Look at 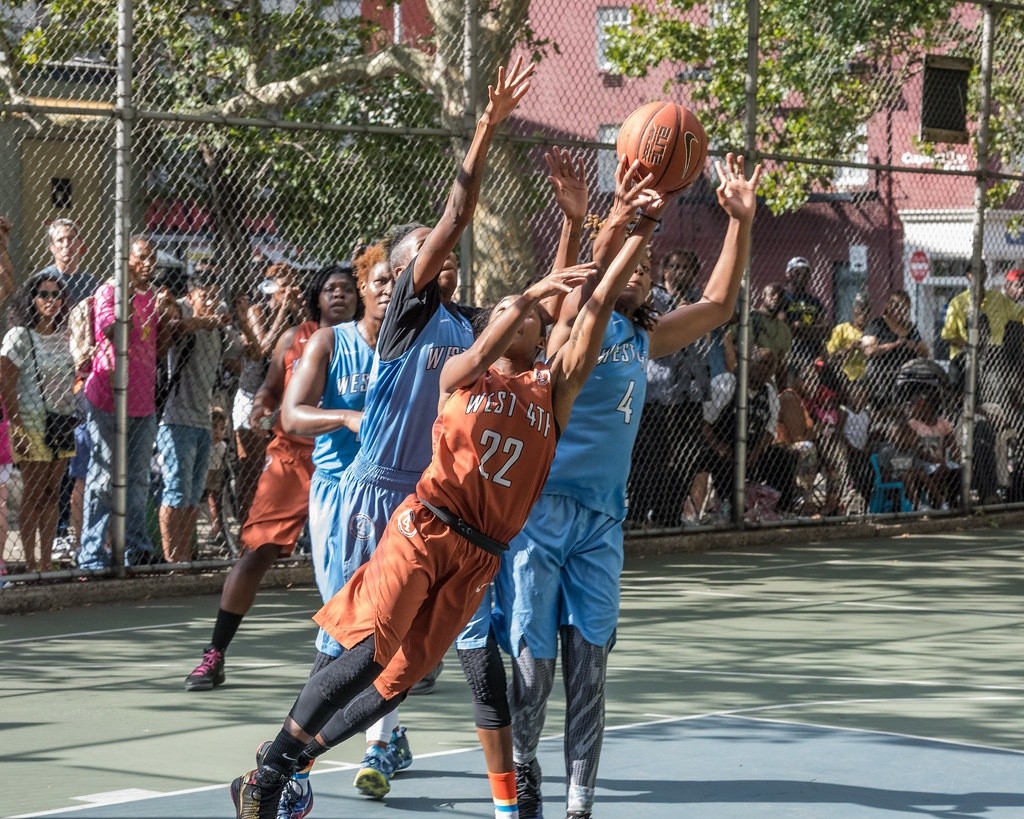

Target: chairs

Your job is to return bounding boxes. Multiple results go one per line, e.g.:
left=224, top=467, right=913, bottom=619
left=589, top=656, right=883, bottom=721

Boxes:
left=868, top=454, right=913, bottom=512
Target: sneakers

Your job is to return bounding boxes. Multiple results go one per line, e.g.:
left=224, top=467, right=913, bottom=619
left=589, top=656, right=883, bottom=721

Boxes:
left=184, top=646, right=226, bottom=690
left=409, top=661, right=443, bottom=696
left=256, top=740, right=296, bottom=819
left=387, top=727, right=413, bottom=778
left=353, top=745, right=394, bottom=798
left=230, top=768, right=289, bottom=819
left=514, top=757, right=544, bottom=819
left=289, top=771, right=315, bottom=818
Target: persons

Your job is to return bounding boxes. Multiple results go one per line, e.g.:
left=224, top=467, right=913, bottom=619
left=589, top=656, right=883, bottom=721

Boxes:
left=484, top=152, right=762, bottom=819
left=230, top=181, right=694, bottom=818
left=280, top=242, right=413, bottom=798
left=622, top=251, right=1024, bottom=530
left=278, top=55, right=588, bottom=819
left=0, top=219, right=304, bottom=575
left=184, top=268, right=443, bottom=695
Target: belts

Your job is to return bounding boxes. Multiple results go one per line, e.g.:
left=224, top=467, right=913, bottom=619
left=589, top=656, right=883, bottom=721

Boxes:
left=422, top=498, right=510, bottom=557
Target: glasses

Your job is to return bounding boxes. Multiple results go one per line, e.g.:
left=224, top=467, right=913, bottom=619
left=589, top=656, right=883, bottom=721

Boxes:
left=37, top=290, right=63, bottom=299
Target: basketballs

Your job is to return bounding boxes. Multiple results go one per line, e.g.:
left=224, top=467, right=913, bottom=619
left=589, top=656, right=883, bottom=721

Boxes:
left=615, top=101, right=708, bottom=192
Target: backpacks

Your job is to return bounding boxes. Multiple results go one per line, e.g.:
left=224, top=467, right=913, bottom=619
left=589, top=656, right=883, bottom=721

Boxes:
left=69, top=279, right=117, bottom=377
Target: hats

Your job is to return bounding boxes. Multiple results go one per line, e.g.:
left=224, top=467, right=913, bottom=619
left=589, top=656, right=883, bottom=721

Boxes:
left=786, top=255, right=810, bottom=274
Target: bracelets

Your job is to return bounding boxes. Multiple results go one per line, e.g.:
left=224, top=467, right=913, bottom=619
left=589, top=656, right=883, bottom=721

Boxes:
left=640, top=213, right=661, bottom=223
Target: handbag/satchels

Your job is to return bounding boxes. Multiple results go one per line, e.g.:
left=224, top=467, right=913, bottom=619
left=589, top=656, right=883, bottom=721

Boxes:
left=44, top=410, right=80, bottom=451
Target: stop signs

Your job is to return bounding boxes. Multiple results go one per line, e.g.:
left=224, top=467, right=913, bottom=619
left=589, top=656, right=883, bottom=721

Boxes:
left=909, top=251, right=931, bottom=284
